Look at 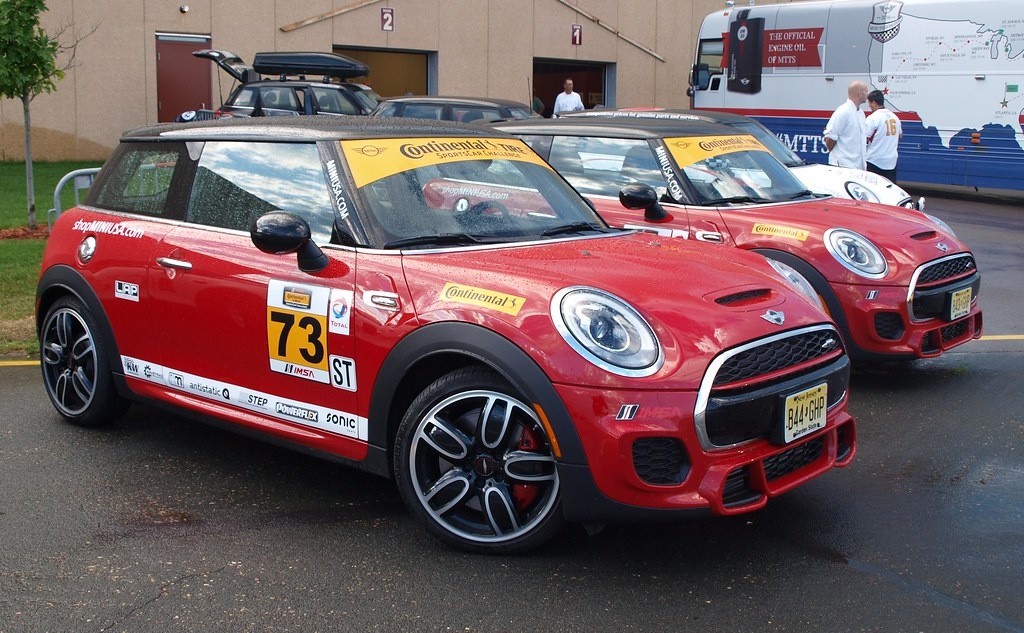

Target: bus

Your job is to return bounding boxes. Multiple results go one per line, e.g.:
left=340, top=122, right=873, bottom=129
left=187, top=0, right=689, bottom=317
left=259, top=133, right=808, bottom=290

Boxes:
left=684, top=1, right=1024, bottom=192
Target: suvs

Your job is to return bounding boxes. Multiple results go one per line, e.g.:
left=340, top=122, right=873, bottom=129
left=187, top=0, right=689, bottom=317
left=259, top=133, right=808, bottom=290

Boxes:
left=33, top=112, right=859, bottom=556
left=556, top=102, right=927, bottom=214
left=173, top=49, right=384, bottom=130
left=469, top=110, right=986, bottom=379
left=368, top=98, right=586, bottom=174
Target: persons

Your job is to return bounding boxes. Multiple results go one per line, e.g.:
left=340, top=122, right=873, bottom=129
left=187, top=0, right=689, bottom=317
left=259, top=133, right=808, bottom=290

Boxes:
left=533, top=88, right=545, bottom=115
left=553, top=78, right=585, bottom=118
left=823, top=80, right=868, bottom=172
left=864, top=90, right=903, bottom=185
